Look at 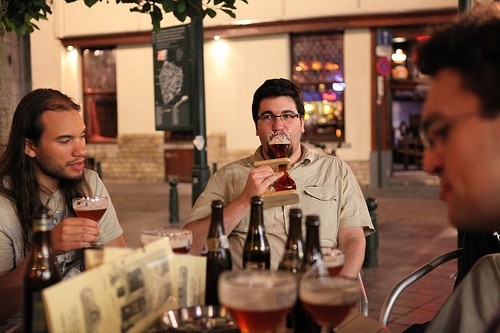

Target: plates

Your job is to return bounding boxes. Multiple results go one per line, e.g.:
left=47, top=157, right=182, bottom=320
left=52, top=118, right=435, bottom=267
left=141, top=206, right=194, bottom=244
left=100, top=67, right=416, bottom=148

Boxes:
left=155, top=304, right=240, bottom=333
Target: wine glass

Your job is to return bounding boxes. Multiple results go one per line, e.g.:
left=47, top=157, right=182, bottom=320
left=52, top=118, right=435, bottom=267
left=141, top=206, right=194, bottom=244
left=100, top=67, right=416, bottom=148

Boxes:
left=71, top=196, right=108, bottom=249
left=267, top=128, right=298, bottom=192
left=299, top=274, right=361, bottom=333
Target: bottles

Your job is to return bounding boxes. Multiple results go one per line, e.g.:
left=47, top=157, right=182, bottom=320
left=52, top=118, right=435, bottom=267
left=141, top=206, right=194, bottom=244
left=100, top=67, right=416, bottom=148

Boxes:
left=242, top=196, right=270, bottom=271
left=276, top=208, right=305, bottom=327
left=299, top=215, right=334, bottom=333
left=23, top=208, right=59, bottom=333
left=201, top=200, right=233, bottom=305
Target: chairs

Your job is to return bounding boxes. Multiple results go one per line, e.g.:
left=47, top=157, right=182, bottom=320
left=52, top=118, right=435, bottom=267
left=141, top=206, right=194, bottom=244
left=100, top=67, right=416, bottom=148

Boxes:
left=379, top=233, right=500, bottom=333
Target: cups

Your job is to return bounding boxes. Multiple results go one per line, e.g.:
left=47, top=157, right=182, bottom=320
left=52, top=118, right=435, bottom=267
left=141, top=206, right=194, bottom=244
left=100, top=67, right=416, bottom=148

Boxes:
left=218, top=270, right=298, bottom=333
left=141, top=226, right=193, bottom=254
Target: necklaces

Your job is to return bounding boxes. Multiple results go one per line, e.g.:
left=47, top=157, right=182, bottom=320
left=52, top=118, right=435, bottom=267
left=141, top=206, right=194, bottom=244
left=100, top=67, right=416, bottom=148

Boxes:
left=46, top=192, right=62, bottom=225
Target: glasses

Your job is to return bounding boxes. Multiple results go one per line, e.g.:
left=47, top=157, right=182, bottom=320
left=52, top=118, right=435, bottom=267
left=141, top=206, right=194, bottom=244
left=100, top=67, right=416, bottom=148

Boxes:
left=257, top=113, right=301, bottom=124
left=418, top=110, right=492, bottom=148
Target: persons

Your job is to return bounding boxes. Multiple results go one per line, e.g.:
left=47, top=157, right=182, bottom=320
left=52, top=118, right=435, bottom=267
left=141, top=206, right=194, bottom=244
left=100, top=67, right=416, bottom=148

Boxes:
left=395, top=120, right=413, bottom=146
left=183, top=78, right=377, bottom=279
left=0, top=87, right=128, bottom=333
left=415, top=1, right=500, bottom=333
